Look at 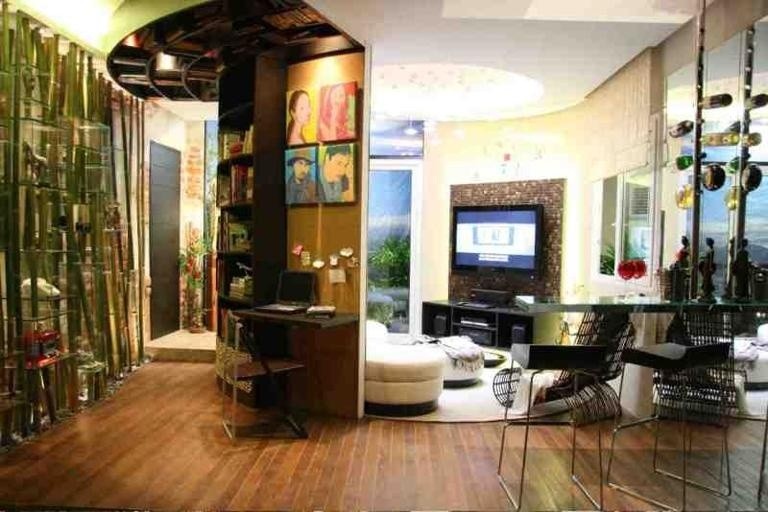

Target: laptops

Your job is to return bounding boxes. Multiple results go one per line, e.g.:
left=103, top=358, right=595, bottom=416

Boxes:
left=255, top=271, right=317, bottom=313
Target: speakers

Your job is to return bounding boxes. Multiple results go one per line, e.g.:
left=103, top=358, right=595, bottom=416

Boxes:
left=511, top=324, right=526, bottom=344
left=433, top=313, right=448, bottom=336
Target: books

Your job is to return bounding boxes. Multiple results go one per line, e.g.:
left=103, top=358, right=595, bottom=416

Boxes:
left=216, top=124, right=253, bottom=300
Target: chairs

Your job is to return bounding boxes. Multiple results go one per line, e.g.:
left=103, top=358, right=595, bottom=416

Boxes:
left=692, top=319, right=768, bottom=391
left=217, top=311, right=311, bottom=442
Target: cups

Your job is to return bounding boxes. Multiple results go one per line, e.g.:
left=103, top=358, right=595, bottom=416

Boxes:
left=660, top=270, right=686, bottom=301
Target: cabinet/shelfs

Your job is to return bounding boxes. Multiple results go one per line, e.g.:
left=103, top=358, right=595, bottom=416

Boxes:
left=217, top=49, right=287, bottom=341
left=421, top=298, right=535, bottom=347
left=1, top=69, right=115, bottom=446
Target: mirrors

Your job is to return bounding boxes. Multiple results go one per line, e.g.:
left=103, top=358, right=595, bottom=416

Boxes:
left=591, top=163, right=654, bottom=289
left=650, top=12, right=768, bottom=423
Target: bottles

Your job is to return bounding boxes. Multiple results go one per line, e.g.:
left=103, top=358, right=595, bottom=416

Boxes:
left=745, top=93, right=768, bottom=111
left=698, top=119, right=763, bottom=175
left=668, top=119, right=704, bottom=138
left=667, top=151, right=708, bottom=174
left=698, top=94, right=732, bottom=109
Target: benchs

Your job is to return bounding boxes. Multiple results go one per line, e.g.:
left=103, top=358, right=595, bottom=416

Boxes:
left=365, top=317, right=445, bottom=416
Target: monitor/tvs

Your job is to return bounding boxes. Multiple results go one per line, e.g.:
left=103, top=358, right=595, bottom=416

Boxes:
left=453, top=205, right=544, bottom=272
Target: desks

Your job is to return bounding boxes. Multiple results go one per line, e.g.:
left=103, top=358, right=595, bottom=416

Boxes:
left=231, top=304, right=359, bottom=330
left=517, top=292, right=768, bottom=316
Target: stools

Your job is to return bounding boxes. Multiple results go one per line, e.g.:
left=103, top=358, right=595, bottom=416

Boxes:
left=420, top=342, right=483, bottom=387
left=602, top=340, right=735, bottom=512
left=498, top=339, right=610, bottom=510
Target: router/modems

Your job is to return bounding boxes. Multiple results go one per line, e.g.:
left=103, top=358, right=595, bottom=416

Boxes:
left=306, top=305, right=337, bottom=317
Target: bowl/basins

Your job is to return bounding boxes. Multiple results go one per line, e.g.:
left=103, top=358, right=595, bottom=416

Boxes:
left=482, top=352, right=506, bottom=368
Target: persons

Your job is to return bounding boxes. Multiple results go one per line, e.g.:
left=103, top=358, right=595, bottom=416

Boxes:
left=318, top=145, right=352, bottom=203
left=318, top=84, right=354, bottom=141
left=733, top=239, right=751, bottom=302
left=286, top=149, right=316, bottom=203
left=286, top=90, right=311, bottom=146
left=677, top=236, right=689, bottom=260
left=698, top=238, right=716, bottom=303
